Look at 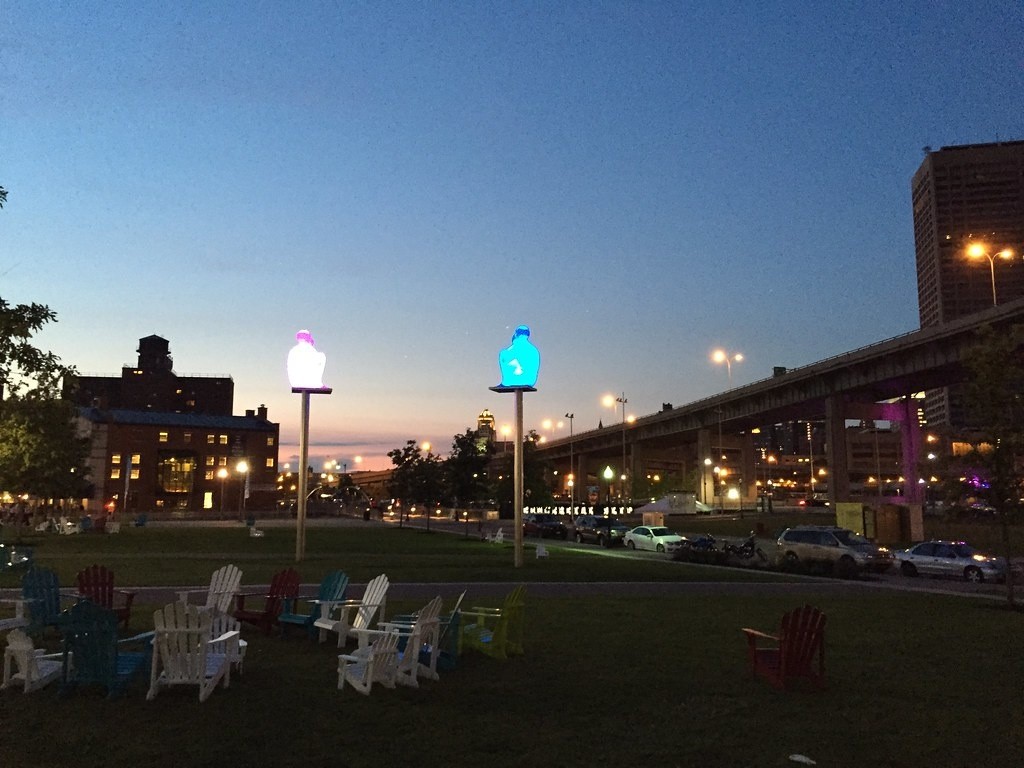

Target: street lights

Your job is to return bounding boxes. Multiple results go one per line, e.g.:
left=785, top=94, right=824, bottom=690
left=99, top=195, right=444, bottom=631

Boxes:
left=968, top=242, right=1014, bottom=309
left=288, top=328, right=332, bottom=564
left=715, top=349, right=742, bottom=392
left=565, top=413, right=574, bottom=524
left=488, top=325, right=541, bottom=570
left=614, top=391, right=628, bottom=520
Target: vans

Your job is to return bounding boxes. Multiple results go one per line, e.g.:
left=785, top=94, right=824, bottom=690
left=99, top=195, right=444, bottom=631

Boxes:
left=776, top=523, right=876, bottom=577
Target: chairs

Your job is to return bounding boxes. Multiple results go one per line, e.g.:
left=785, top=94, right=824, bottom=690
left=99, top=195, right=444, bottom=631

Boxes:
left=145, top=603, right=236, bottom=700
left=277, top=569, right=349, bottom=642
left=77, top=564, right=139, bottom=625
left=336, top=631, right=400, bottom=695
left=54, top=598, right=148, bottom=699
left=313, top=572, right=390, bottom=648
left=353, top=595, right=444, bottom=690
left=392, top=587, right=470, bottom=670
left=450, top=582, right=530, bottom=661
left=198, top=608, right=249, bottom=674
left=536, top=543, right=550, bottom=560
left=486, top=533, right=495, bottom=543
left=22, top=566, right=92, bottom=638
left=36, top=511, right=148, bottom=534
left=0, top=628, right=73, bottom=693
left=176, top=564, right=244, bottom=630
left=742, top=606, right=828, bottom=692
left=232, top=568, right=300, bottom=640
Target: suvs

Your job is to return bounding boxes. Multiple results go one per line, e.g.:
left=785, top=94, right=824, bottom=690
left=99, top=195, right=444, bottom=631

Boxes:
left=574, top=516, right=633, bottom=547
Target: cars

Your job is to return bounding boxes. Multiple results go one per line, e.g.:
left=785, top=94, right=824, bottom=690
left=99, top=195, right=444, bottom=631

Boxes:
left=522, top=513, right=569, bottom=541
left=875, top=540, right=897, bottom=573
left=892, top=539, right=1007, bottom=585
left=624, top=525, right=692, bottom=554
